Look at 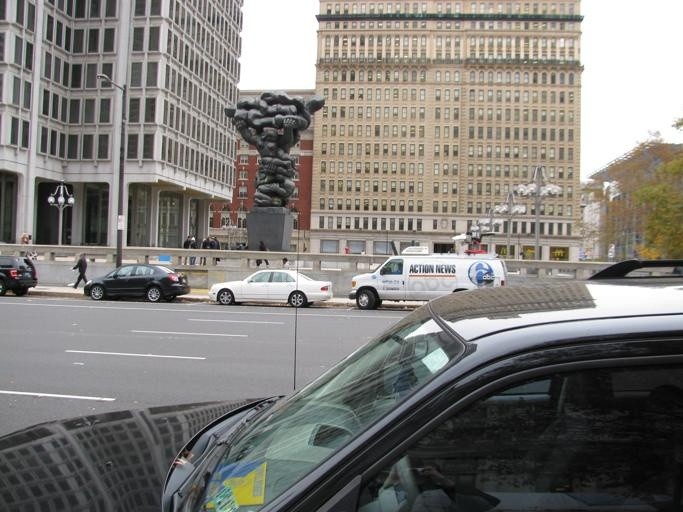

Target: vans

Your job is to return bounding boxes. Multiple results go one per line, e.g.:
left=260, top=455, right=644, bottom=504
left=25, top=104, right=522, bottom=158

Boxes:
left=349, top=245, right=508, bottom=310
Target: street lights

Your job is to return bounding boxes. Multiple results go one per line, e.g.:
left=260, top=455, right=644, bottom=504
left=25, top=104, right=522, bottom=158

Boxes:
left=47, top=180, right=75, bottom=245
left=472, top=192, right=527, bottom=259
left=221, top=218, right=248, bottom=250
left=515, top=164, right=563, bottom=259
left=97, top=73, right=127, bottom=268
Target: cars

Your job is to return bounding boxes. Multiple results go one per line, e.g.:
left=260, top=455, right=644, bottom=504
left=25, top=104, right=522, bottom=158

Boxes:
left=209, top=269, right=332, bottom=308
left=84, top=264, right=190, bottom=302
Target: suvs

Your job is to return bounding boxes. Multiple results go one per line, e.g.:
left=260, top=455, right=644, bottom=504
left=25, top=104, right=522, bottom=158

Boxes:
left=0, top=256, right=37, bottom=296
left=0, top=258, right=683, bottom=511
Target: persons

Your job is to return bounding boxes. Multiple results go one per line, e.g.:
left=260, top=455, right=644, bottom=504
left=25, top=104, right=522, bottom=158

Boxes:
left=281, top=258, right=289, bottom=270
left=184, top=235, right=249, bottom=265
left=26, top=235, right=34, bottom=260
left=72, top=253, right=88, bottom=289
left=257, top=241, right=270, bottom=268
left=385, top=263, right=402, bottom=274
left=20, top=233, right=28, bottom=257
